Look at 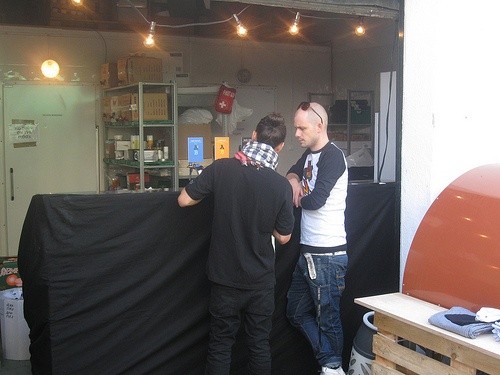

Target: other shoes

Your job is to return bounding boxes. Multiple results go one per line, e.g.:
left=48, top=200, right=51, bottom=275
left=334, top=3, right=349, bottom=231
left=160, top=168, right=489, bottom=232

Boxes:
left=316, top=365, right=346, bottom=375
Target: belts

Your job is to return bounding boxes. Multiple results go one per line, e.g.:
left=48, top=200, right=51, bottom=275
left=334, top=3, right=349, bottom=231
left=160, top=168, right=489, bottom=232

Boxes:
left=299, top=250, right=347, bottom=280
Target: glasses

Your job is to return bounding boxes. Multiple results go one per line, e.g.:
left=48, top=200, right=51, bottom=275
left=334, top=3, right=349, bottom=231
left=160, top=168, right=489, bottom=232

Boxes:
left=296, top=101, right=323, bottom=123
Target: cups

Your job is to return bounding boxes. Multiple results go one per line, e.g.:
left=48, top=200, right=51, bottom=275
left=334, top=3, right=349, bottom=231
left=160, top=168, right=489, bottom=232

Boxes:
left=114, top=135, right=124, bottom=159
left=130, top=135, right=140, bottom=149
left=147, top=136, right=154, bottom=149
left=105, top=139, right=115, bottom=159
left=134, top=151, right=154, bottom=162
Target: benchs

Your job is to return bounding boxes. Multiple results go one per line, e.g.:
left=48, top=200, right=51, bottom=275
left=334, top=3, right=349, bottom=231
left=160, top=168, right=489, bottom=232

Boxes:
left=354, top=161, right=500, bottom=375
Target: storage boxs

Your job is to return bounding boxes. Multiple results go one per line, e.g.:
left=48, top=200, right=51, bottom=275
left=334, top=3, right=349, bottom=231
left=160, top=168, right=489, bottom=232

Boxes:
left=178, top=123, right=213, bottom=160
left=101, top=56, right=168, bottom=121
left=0, top=258, right=23, bottom=291
left=114, top=140, right=173, bottom=191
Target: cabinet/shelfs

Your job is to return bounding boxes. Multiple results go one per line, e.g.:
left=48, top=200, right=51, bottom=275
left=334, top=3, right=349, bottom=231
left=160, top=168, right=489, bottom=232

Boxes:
left=100, top=83, right=178, bottom=193
left=307, top=88, right=375, bottom=186
left=177, top=80, right=228, bottom=188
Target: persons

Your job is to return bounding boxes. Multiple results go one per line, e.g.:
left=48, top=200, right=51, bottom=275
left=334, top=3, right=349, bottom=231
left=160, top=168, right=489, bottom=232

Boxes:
left=178, top=113, right=295, bottom=375
left=286, top=102, right=349, bottom=375
